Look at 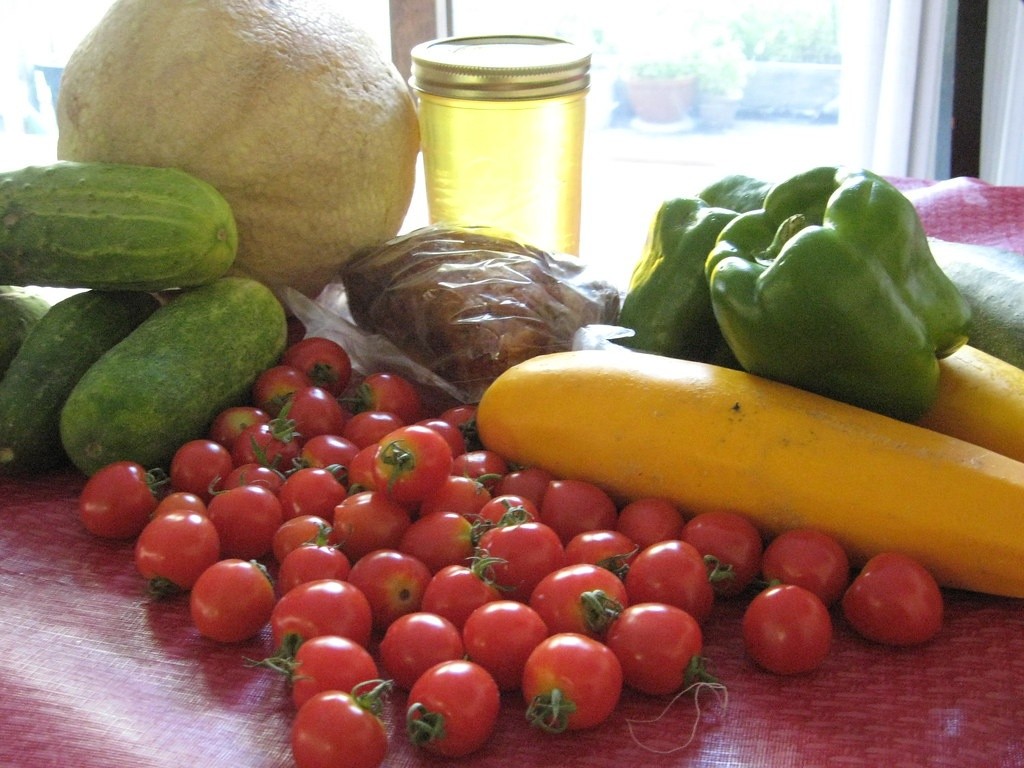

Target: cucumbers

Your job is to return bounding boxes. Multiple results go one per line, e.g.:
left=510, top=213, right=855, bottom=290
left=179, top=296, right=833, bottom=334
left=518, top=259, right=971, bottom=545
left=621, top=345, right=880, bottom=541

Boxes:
left=0, top=161, right=288, bottom=477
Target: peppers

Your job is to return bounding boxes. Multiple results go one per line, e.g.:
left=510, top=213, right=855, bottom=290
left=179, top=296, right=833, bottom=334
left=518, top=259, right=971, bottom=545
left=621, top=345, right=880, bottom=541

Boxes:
left=616, top=165, right=970, bottom=421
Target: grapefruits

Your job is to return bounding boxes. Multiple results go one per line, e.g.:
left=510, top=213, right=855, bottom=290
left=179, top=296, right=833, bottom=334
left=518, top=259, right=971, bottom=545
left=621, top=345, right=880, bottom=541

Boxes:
left=51, top=1, right=427, bottom=307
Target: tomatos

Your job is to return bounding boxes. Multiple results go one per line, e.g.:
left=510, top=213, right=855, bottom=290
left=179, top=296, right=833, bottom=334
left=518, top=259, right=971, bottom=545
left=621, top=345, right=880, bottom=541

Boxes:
left=80, top=337, right=942, bottom=768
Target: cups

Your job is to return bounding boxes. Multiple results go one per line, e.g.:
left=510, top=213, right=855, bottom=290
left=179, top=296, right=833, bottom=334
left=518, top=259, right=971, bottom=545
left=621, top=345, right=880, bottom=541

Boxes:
left=408, top=32, right=594, bottom=265
